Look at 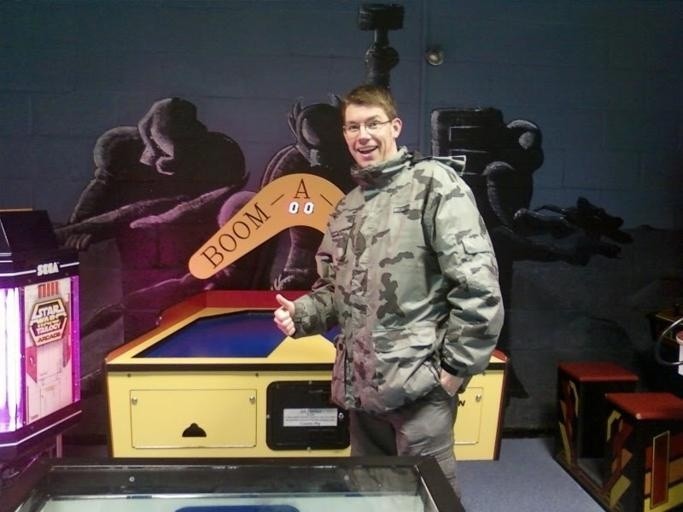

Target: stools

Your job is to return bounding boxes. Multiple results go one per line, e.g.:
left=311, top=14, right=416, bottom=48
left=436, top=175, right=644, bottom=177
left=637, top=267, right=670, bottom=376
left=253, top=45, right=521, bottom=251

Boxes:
left=552, top=353, right=681, bottom=512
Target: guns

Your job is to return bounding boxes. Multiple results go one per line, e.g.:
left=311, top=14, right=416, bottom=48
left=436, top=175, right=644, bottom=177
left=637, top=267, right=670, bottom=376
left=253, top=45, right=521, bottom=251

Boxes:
left=513, top=198, right=634, bottom=258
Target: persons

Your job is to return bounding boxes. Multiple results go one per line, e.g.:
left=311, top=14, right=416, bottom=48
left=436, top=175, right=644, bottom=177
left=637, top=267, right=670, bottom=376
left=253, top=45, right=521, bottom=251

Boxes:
left=272, top=84, right=506, bottom=512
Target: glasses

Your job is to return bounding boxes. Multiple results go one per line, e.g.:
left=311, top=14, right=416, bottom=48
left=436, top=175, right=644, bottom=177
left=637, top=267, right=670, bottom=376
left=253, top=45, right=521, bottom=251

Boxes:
left=344, top=120, right=391, bottom=138
